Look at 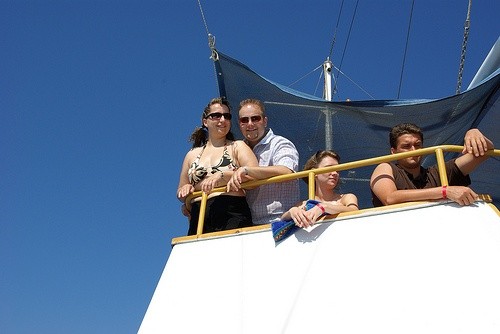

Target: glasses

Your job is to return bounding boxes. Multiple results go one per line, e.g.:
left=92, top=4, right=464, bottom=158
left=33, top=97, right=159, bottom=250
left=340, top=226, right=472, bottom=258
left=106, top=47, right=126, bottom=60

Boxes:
left=238, top=115, right=266, bottom=123
left=205, top=112, right=232, bottom=120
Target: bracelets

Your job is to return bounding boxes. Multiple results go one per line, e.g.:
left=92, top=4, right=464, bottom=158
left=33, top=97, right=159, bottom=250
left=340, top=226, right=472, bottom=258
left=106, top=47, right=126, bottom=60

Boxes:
left=220, top=171, right=225, bottom=180
left=243, top=167, right=248, bottom=175
left=316, top=204, right=325, bottom=214
left=442, top=186, right=446, bottom=200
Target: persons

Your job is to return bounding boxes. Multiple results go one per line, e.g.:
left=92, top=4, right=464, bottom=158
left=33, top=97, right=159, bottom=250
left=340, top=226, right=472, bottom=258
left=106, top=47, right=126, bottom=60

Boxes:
left=280, top=151, right=358, bottom=229
left=369, top=123, right=494, bottom=207
left=176, top=99, right=259, bottom=236
left=181, top=99, right=300, bottom=226
